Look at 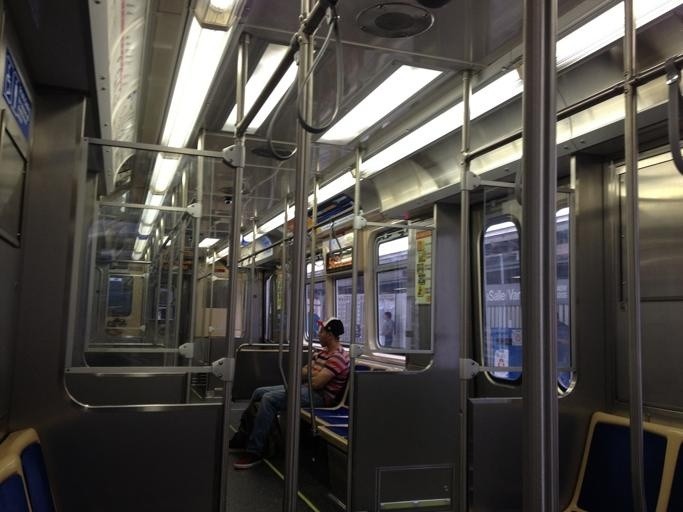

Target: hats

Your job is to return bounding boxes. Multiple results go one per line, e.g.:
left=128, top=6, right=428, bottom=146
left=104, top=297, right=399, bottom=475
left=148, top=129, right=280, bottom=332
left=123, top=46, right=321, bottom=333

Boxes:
left=316, top=317, right=344, bottom=337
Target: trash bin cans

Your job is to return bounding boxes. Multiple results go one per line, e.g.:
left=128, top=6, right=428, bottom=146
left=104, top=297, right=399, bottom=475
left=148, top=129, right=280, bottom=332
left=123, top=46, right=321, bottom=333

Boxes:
left=492, top=327, right=523, bottom=381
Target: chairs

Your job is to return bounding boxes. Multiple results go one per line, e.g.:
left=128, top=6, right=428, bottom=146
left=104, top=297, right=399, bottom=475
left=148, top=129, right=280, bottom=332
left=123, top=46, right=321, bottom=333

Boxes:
left=299, top=356, right=405, bottom=452
left=559, top=410, right=682, bottom=507
left=0, top=425, right=61, bottom=510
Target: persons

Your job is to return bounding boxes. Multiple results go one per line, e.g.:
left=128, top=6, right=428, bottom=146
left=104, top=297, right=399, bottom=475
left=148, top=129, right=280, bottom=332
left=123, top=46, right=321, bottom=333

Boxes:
left=230, top=317, right=351, bottom=469
left=380, top=311, right=393, bottom=346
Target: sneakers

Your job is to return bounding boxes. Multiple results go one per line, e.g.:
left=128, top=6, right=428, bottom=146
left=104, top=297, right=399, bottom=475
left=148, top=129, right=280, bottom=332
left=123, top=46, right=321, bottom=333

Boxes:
left=228, top=434, right=262, bottom=469
